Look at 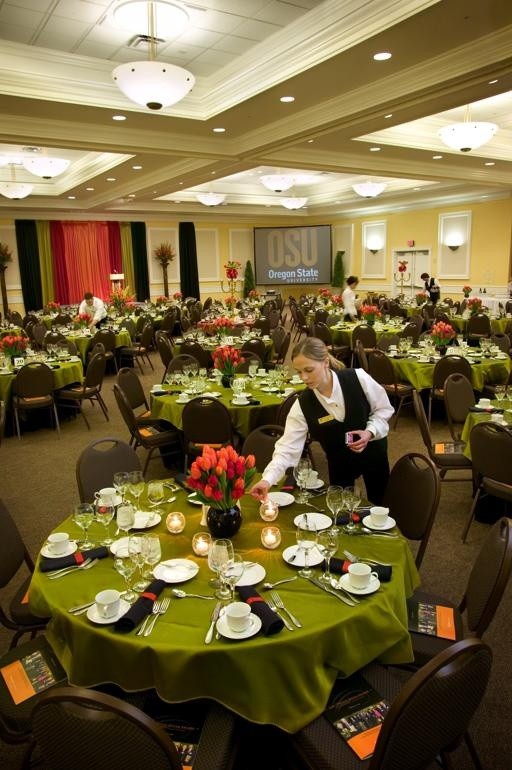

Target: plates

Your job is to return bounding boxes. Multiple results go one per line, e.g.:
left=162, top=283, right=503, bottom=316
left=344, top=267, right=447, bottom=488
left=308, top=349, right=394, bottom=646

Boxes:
left=213, top=612, right=263, bottom=642
left=338, top=571, right=383, bottom=598
left=361, top=514, right=396, bottom=531
left=230, top=398, right=251, bottom=405
left=86, top=599, right=131, bottom=626
left=472, top=404, right=494, bottom=410
left=175, top=398, right=193, bottom=404
left=39, top=541, right=77, bottom=559
left=485, top=420, right=509, bottom=426
left=288, top=379, right=305, bottom=384
left=150, top=390, right=166, bottom=393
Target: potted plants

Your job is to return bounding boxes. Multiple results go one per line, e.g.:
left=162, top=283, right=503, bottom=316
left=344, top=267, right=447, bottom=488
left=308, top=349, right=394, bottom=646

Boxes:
left=331, top=250, right=345, bottom=296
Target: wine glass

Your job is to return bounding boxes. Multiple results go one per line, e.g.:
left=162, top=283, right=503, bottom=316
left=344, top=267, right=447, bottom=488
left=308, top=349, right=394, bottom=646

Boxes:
left=0, top=342, right=69, bottom=368
left=495, top=384, right=512, bottom=413
left=167, top=361, right=290, bottom=402
left=73, top=457, right=364, bottom=607
left=395, top=334, right=508, bottom=365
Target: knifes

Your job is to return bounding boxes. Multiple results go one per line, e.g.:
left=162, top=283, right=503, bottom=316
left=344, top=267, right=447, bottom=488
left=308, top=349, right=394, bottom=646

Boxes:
left=204, top=598, right=221, bottom=649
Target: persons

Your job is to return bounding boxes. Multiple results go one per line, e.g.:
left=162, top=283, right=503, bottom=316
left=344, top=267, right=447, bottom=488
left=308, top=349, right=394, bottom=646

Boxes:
left=250, top=336, right=396, bottom=508
left=78, top=292, right=107, bottom=330
left=342, top=276, right=360, bottom=322
left=421, top=273, right=441, bottom=306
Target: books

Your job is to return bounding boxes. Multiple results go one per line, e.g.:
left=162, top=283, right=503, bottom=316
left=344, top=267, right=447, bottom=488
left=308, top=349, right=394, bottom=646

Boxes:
left=140, top=698, right=210, bottom=770
left=22, top=396, right=46, bottom=401
left=138, top=422, right=174, bottom=438
left=0, top=647, right=68, bottom=706
left=141, top=410, right=152, bottom=417
left=389, top=383, right=407, bottom=391
left=322, top=671, right=392, bottom=761
left=406, top=599, right=456, bottom=640
left=434, top=443, right=466, bottom=455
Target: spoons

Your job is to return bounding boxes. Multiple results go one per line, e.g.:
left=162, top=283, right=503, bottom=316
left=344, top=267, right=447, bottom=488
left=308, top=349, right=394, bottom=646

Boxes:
left=358, top=527, right=398, bottom=538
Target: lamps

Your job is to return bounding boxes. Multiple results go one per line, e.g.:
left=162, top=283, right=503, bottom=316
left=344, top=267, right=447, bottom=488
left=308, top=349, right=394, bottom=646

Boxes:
left=438, top=103, right=499, bottom=154
left=110, top=0, right=196, bottom=113
left=195, top=173, right=388, bottom=211
left=367, top=233, right=384, bottom=255
left=444, top=230, right=465, bottom=251
left=0, top=150, right=34, bottom=203
left=24, top=145, right=71, bottom=184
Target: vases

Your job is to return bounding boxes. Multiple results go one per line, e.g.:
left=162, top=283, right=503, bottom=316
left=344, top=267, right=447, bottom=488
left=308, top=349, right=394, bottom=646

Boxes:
left=206, top=507, right=243, bottom=538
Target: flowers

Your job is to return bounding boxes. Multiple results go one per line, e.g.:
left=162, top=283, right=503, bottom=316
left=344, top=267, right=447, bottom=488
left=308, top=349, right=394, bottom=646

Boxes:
left=398, top=260, right=409, bottom=272
left=462, top=286, right=472, bottom=294
left=223, top=260, right=243, bottom=280
left=103, top=283, right=135, bottom=309
left=248, top=289, right=258, bottom=298
left=152, top=238, right=177, bottom=263
left=187, top=444, right=263, bottom=512
left=317, top=288, right=328, bottom=295
left=0, top=241, right=14, bottom=267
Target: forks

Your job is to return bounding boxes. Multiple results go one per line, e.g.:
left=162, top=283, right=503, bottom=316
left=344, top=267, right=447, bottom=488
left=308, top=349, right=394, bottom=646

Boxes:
left=265, top=591, right=303, bottom=632
left=135, top=597, right=172, bottom=642
left=45, top=556, right=100, bottom=580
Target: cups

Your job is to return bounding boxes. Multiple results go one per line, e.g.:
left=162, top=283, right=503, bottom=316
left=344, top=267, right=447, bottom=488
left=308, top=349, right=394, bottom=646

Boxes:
left=96, top=589, right=121, bottom=617
left=368, top=505, right=391, bottom=527
left=347, top=562, right=379, bottom=589
left=47, top=533, right=69, bottom=555
left=153, top=384, right=162, bottom=391
left=293, top=375, right=300, bottom=381
left=478, top=399, right=492, bottom=408
left=285, top=387, right=298, bottom=398
left=492, top=413, right=504, bottom=424
left=223, top=601, right=253, bottom=631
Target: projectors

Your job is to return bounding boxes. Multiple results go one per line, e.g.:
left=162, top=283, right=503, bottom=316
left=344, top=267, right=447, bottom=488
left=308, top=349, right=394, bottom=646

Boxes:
left=266, top=290, right=276, bottom=294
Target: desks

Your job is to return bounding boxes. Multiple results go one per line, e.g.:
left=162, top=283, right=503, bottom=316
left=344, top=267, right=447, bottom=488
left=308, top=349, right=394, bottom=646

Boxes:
left=29, top=473, right=419, bottom=732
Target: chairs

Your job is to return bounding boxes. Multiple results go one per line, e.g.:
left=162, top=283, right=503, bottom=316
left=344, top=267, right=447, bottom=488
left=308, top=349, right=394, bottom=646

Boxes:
left=242, top=426, right=305, bottom=479
left=384, top=517, right=512, bottom=668
left=1, top=293, right=511, bottom=479
left=77, top=438, right=142, bottom=503
left=0, top=501, right=49, bottom=654
left=382, top=452, right=441, bottom=571
left=0, top=635, right=69, bottom=744
left=460, top=421, right=512, bottom=542
left=290, top=640, right=495, bottom=768
left=17, top=688, right=235, bottom=770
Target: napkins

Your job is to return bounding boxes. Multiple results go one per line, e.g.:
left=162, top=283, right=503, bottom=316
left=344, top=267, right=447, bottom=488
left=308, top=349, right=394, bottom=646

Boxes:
left=282, top=475, right=296, bottom=511
left=234, top=586, right=285, bottom=636
left=39, top=546, right=108, bottom=573
left=113, top=579, right=165, bottom=632
left=175, top=475, right=193, bottom=494
left=322, top=558, right=394, bottom=581
left=335, top=510, right=371, bottom=525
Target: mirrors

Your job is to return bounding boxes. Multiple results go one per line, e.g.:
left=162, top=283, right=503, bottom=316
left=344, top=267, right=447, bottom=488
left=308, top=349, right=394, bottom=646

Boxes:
left=359, top=219, right=388, bottom=280
left=436, top=209, right=472, bottom=280
